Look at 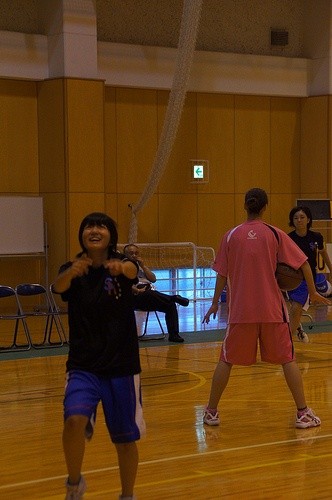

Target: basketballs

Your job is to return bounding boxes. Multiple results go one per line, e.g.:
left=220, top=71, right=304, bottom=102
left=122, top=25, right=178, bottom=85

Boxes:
left=275, top=263, right=303, bottom=290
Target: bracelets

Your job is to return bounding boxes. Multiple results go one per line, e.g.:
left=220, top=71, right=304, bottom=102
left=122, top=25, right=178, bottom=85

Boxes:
left=212, top=300, right=219, bottom=303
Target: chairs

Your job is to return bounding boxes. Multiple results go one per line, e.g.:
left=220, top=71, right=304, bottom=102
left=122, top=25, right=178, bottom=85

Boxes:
left=0, top=283, right=165, bottom=353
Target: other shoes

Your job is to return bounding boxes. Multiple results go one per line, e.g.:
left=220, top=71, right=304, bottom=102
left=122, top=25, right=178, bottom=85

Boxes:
left=64, top=474, right=86, bottom=500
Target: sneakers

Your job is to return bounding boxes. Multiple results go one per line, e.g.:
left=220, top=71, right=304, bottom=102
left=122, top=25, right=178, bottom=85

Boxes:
left=203, top=408, right=220, bottom=425
left=296, top=408, right=321, bottom=428
left=296, top=323, right=309, bottom=344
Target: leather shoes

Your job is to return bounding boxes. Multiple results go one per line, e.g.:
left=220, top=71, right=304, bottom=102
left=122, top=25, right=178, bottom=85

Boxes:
left=174, top=295, right=189, bottom=306
left=168, top=335, right=184, bottom=343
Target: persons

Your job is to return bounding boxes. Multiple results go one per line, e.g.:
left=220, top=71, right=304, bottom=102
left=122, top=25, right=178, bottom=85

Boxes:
left=276, top=206, right=332, bottom=343
left=123, top=244, right=189, bottom=344
left=202, top=187, right=330, bottom=428
left=51, top=212, right=156, bottom=500
